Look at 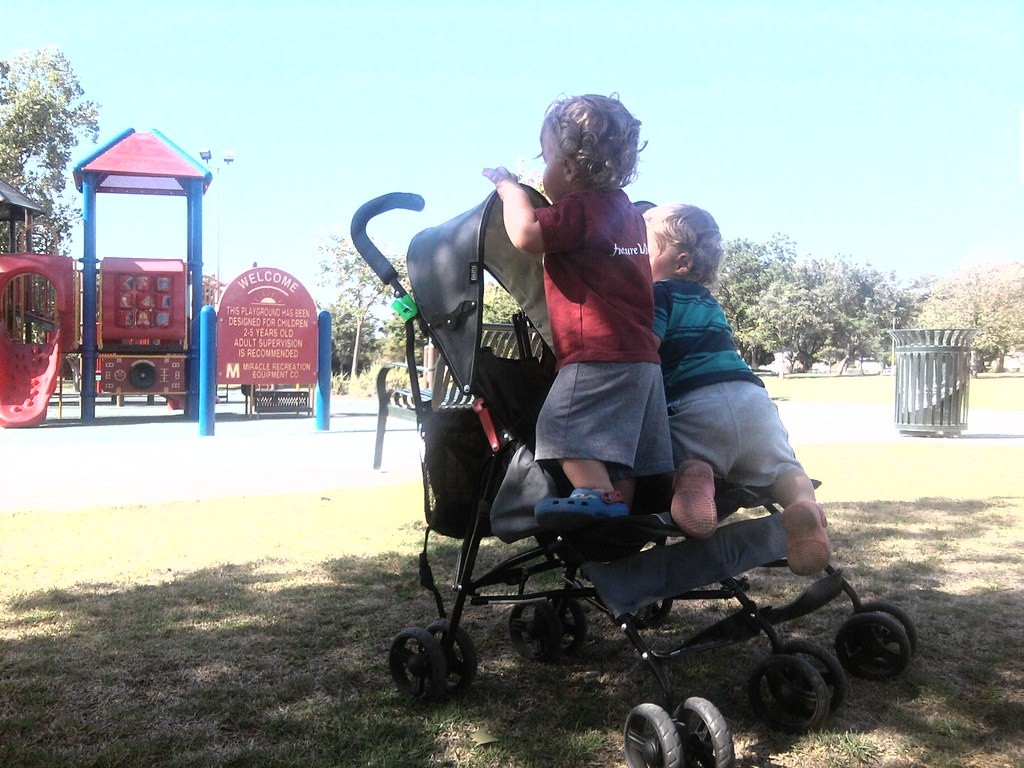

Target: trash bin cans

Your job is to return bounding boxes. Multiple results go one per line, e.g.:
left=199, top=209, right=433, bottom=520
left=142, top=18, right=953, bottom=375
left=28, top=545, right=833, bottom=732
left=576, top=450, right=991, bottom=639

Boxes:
left=886, top=327, right=982, bottom=438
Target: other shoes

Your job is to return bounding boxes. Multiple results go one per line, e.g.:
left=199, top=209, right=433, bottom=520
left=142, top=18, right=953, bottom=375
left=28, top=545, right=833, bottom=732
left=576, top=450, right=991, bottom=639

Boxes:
left=671, top=460, right=717, bottom=539
left=782, top=499, right=831, bottom=577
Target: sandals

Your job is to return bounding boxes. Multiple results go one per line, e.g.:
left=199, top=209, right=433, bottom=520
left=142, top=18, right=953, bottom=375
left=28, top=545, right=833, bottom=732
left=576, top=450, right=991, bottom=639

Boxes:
left=534, top=489, right=629, bottom=520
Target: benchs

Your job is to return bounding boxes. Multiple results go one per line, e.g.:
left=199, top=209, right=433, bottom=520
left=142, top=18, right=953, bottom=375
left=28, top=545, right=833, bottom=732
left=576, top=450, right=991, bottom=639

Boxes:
left=372, top=325, right=542, bottom=470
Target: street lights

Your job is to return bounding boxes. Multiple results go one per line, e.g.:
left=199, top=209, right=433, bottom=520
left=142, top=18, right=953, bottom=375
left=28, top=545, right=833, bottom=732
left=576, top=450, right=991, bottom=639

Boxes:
left=200, top=149, right=235, bottom=306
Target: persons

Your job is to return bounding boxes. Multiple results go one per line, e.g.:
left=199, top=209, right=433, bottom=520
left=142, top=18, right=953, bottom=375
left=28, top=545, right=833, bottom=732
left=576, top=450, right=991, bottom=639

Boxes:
left=480, top=92, right=676, bottom=525
left=633, top=200, right=833, bottom=575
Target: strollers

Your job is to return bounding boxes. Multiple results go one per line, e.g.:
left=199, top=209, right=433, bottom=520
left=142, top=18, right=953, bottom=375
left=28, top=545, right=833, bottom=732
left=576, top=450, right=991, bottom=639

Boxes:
left=349, top=184, right=917, bottom=767
